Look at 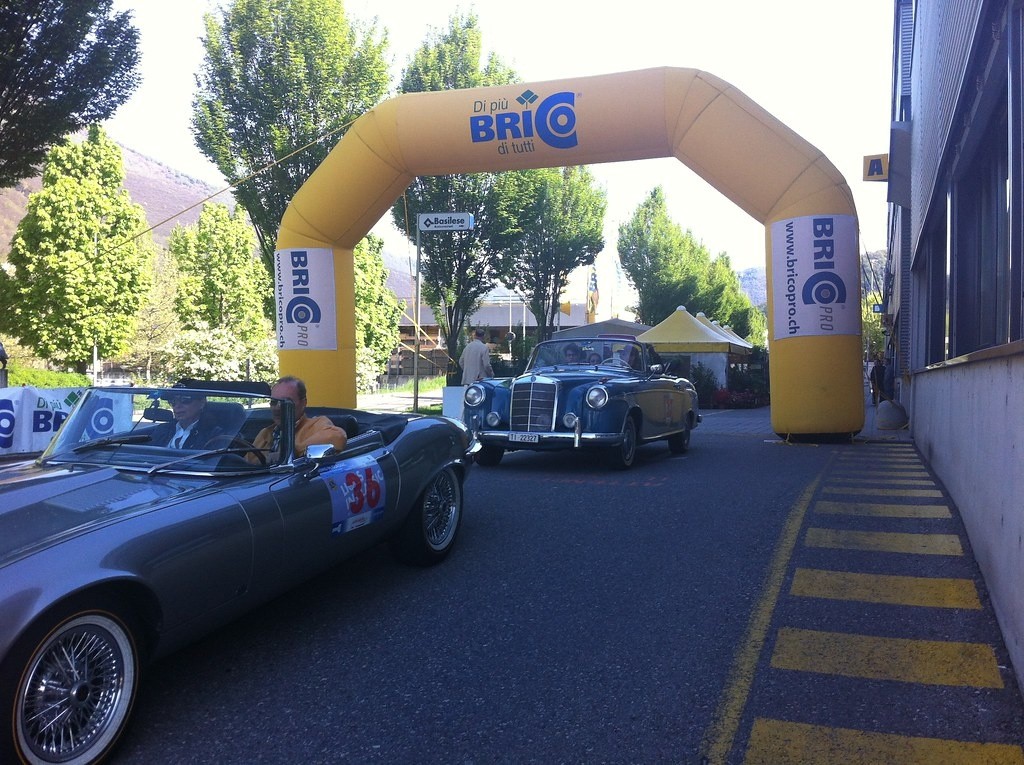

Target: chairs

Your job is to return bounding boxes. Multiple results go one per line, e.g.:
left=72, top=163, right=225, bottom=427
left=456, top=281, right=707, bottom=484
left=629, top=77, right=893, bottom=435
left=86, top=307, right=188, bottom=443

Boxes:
left=201, top=402, right=248, bottom=453
left=317, top=414, right=361, bottom=441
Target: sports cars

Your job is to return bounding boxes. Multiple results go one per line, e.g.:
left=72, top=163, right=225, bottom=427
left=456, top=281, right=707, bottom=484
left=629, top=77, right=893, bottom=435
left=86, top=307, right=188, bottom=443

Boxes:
left=0, top=378, right=485, bottom=765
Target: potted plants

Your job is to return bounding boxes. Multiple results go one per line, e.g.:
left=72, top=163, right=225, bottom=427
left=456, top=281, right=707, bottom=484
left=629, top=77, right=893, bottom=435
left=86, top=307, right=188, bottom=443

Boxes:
left=688, top=344, right=771, bottom=409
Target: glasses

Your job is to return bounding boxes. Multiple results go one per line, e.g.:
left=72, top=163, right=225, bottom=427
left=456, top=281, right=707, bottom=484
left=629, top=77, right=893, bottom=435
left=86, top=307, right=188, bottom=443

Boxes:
left=567, top=354, right=578, bottom=357
left=268, top=398, right=302, bottom=406
left=590, top=359, right=600, bottom=362
left=167, top=395, right=197, bottom=406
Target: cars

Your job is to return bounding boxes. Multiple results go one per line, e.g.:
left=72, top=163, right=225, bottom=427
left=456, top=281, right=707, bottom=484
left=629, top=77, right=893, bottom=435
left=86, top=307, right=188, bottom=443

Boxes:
left=460, top=337, right=705, bottom=468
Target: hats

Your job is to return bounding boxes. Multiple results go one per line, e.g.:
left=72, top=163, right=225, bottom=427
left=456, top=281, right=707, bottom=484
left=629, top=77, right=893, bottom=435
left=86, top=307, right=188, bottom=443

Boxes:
left=161, top=379, right=204, bottom=400
left=617, top=346, right=639, bottom=356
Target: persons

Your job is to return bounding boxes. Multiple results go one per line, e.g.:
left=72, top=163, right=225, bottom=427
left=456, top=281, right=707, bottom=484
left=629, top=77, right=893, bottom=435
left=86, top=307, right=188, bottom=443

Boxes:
left=244, top=375, right=347, bottom=466
left=870, top=359, right=886, bottom=406
left=564, top=344, right=582, bottom=364
left=616, top=345, right=641, bottom=370
left=459, top=329, right=495, bottom=386
left=590, top=353, right=602, bottom=365
left=144, top=377, right=227, bottom=452
left=883, top=358, right=894, bottom=401
